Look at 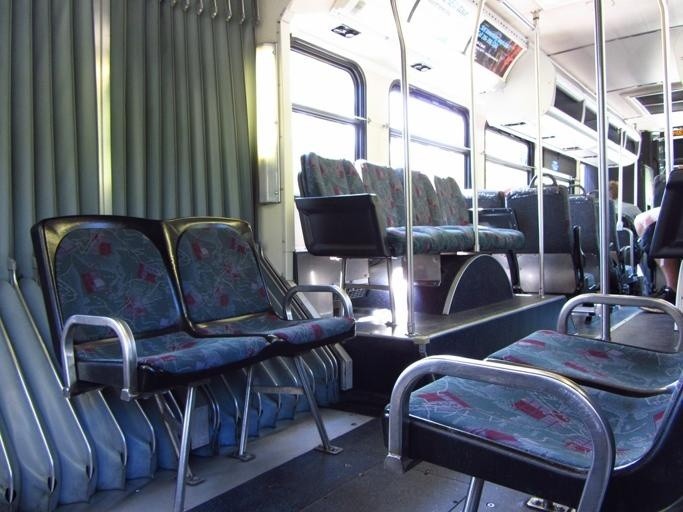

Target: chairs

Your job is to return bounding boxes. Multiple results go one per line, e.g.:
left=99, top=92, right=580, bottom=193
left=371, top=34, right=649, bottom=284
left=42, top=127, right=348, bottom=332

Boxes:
left=460, top=188, right=506, bottom=209
left=508, top=174, right=576, bottom=254
left=567, top=186, right=600, bottom=255
left=31, top=215, right=290, bottom=511
left=400, top=168, right=506, bottom=252
left=355, top=158, right=458, bottom=252
left=587, top=189, right=620, bottom=252
left=488, top=292, right=683, bottom=394
left=301, top=150, right=432, bottom=256
left=164, top=217, right=356, bottom=459
left=382, top=355, right=683, bottom=508
left=435, top=175, right=526, bottom=252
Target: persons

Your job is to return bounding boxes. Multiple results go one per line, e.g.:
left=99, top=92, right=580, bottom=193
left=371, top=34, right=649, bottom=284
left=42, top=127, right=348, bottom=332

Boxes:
left=608, top=180, right=642, bottom=220
left=635, top=206, right=679, bottom=313
